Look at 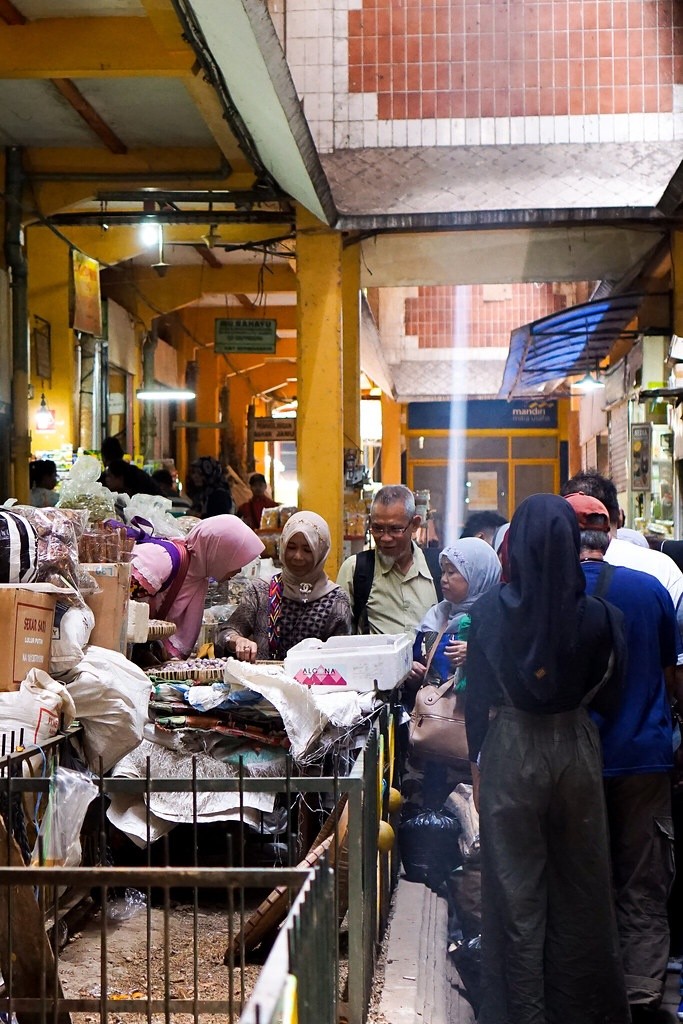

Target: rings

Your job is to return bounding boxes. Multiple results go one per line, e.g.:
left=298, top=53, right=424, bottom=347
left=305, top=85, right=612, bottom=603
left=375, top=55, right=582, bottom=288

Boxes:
left=244, top=646, right=249, bottom=648
left=458, top=657, right=460, bottom=663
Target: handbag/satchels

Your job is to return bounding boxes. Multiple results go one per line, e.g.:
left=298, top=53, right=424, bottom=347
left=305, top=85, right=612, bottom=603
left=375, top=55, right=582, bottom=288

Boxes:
left=408, top=682, right=470, bottom=762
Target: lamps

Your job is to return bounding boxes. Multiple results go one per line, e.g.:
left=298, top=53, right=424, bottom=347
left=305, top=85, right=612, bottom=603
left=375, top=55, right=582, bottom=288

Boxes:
left=134, top=387, right=196, bottom=402
left=571, top=319, right=606, bottom=389
left=35, top=376, right=54, bottom=429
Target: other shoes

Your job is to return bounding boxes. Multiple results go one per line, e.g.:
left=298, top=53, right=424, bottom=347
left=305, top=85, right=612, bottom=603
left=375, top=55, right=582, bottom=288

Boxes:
left=667, top=957, right=683, bottom=969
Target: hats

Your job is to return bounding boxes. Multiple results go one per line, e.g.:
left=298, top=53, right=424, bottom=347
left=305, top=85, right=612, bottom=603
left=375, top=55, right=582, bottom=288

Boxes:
left=562, top=492, right=611, bottom=535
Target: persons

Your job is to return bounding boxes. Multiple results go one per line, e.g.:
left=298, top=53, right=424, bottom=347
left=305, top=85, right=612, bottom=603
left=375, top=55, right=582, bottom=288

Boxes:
left=335, top=485, right=443, bottom=635
left=561, top=473, right=683, bottom=1024
left=464, top=494, right=624, bottom=1024
left=213, top=511, right=354, bottom=664
left=131, top=513, right=266, bottom=670
left=460, top=512, right=512, bottom=561
left=236, top=473, right=280, bottom=530
left=184, top=457, right=232, bottom=519
left=29, top=460, right=60, bottom=506
left=96, top=436, right=188, bottom=507
left=401, top=537, right=501, bottom=937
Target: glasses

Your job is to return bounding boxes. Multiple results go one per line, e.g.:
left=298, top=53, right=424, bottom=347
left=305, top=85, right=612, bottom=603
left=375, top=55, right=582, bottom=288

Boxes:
left=366, top=512, right=415, bottom=537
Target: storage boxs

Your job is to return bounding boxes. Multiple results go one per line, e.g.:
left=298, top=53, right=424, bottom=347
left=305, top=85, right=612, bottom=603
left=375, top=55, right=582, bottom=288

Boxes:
left=0, top=563, right=148, bottom=693
left=285, top=632, right=417, bottom=692
left=641, top=336, right=674, bottom=390
